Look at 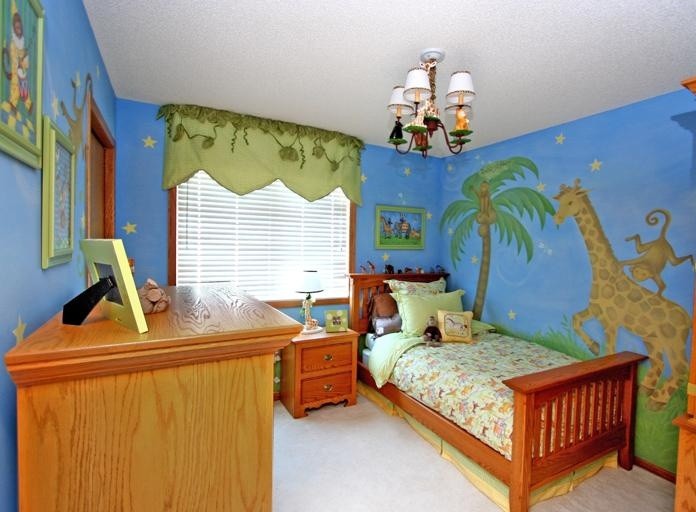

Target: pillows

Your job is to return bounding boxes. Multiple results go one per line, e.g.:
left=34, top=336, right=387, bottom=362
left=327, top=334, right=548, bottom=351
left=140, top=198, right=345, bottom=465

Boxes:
left=371, top=278, right=475, bottom=345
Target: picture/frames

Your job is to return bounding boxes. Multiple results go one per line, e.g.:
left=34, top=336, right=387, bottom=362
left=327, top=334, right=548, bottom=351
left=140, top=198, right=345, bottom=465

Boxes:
left=1, top=0, right=148, bottom=334
left=375, top=204, right=425, bottom=251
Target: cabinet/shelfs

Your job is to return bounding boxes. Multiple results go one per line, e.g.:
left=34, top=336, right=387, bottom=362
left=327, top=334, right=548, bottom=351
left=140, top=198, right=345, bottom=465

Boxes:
left=672, top=412, right=696, bottom=512
left=4, top=284, right=361, bottom=512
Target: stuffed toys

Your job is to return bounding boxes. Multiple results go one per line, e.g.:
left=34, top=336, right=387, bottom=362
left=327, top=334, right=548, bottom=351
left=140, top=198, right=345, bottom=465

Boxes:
left=422, top=316, right=441, bottom=343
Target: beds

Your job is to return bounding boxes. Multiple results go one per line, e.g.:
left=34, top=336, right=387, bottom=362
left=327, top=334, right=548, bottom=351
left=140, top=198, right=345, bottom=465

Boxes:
left=347, top=271, right=650, bottom=512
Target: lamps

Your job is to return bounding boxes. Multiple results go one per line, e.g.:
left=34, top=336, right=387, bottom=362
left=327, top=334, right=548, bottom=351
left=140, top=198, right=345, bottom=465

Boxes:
left=386, top=51, right=476, bottom=158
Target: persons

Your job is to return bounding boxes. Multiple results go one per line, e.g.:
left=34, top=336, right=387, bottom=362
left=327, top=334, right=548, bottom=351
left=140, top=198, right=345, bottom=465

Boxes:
left=7, top=13, right=35, bottom=116
